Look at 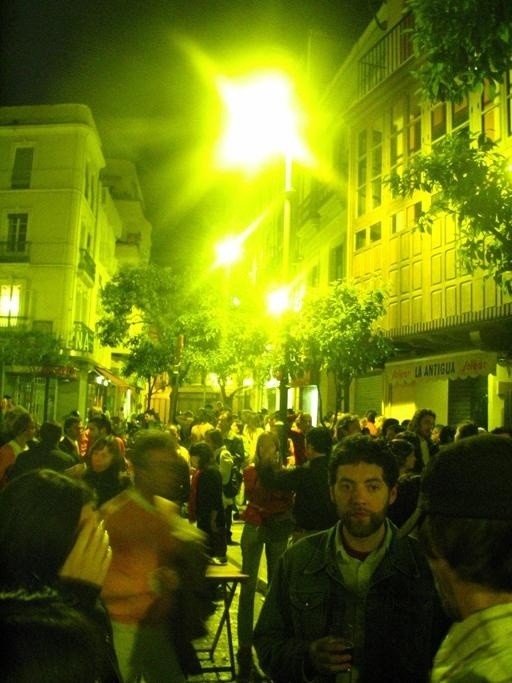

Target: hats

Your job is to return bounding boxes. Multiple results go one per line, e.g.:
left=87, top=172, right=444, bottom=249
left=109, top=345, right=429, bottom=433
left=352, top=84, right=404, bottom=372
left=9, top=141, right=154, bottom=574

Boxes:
left=398, top=433, right=512, bottom=539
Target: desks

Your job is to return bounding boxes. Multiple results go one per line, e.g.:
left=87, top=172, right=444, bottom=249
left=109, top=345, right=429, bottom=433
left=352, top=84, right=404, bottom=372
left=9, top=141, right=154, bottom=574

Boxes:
left=189, top=558, right=256, bottom=682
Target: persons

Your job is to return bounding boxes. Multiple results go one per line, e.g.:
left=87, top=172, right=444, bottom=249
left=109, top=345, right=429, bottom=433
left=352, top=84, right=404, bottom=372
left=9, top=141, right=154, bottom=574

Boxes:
left=0, top=389, right=511, bottom=683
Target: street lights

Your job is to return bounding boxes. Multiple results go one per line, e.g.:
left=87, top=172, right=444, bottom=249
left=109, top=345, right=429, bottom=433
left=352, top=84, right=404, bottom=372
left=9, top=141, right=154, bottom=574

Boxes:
left=210, top=53, right=291, bottom=467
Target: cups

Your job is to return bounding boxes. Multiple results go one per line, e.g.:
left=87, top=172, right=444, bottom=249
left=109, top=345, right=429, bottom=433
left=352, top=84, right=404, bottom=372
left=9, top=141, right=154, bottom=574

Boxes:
left=326, top=621, right=355, bottom=672
